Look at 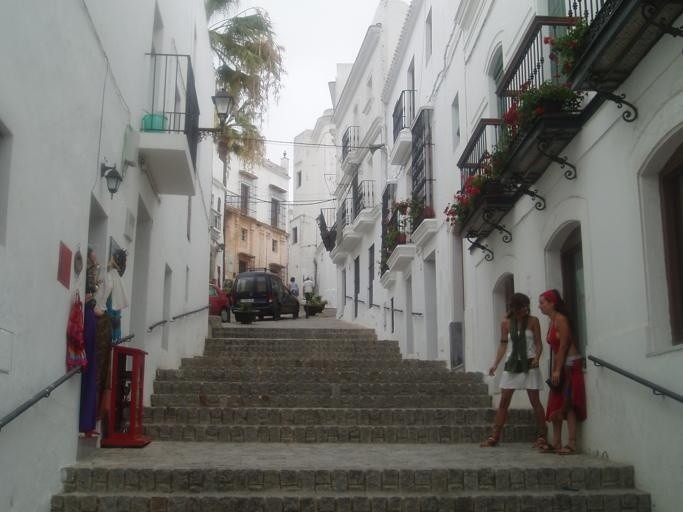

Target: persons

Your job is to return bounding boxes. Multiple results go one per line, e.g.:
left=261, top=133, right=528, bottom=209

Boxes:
left=212, top=278, right=217, bottom=285
left=538, top=289, right=587, bottom=454
left=222, top=278, right=233, bottom=295
left=480, top=292, right=548, bottom=450
left=79, top=249, right=128, bottom=442
left=289, top=276, right=299, bottom=297
left=303, top=277, right=315, bottom=301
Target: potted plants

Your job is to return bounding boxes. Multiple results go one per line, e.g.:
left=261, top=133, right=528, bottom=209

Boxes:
left=304, top=295, right=328, bottom=316
left=232, top=303, right=260, bottom=323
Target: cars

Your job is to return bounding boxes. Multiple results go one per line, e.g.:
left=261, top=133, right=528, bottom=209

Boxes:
left=209, top=283, right=229, bottom=323
left=282, top=286, right=300, bottom=318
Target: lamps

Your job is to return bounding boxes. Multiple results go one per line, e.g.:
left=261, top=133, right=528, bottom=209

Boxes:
left=101, top=163, right=122, bottom=200
left=198, top=88, right=235, bottom=143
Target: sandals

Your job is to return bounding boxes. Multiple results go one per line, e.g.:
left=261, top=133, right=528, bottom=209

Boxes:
left=535, top=423, right=547, bottom=444
left=480, top=435, right=499, bottom=447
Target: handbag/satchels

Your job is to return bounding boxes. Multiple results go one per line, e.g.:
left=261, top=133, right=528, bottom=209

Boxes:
left=545, top=368, right=564, bottom=390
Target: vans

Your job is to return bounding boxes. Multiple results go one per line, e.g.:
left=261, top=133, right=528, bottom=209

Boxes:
left=230, top=271, right=283, bottom=319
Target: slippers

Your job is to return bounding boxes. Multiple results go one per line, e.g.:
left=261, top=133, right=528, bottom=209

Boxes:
left=533, top=440, right=577, bottom=455
